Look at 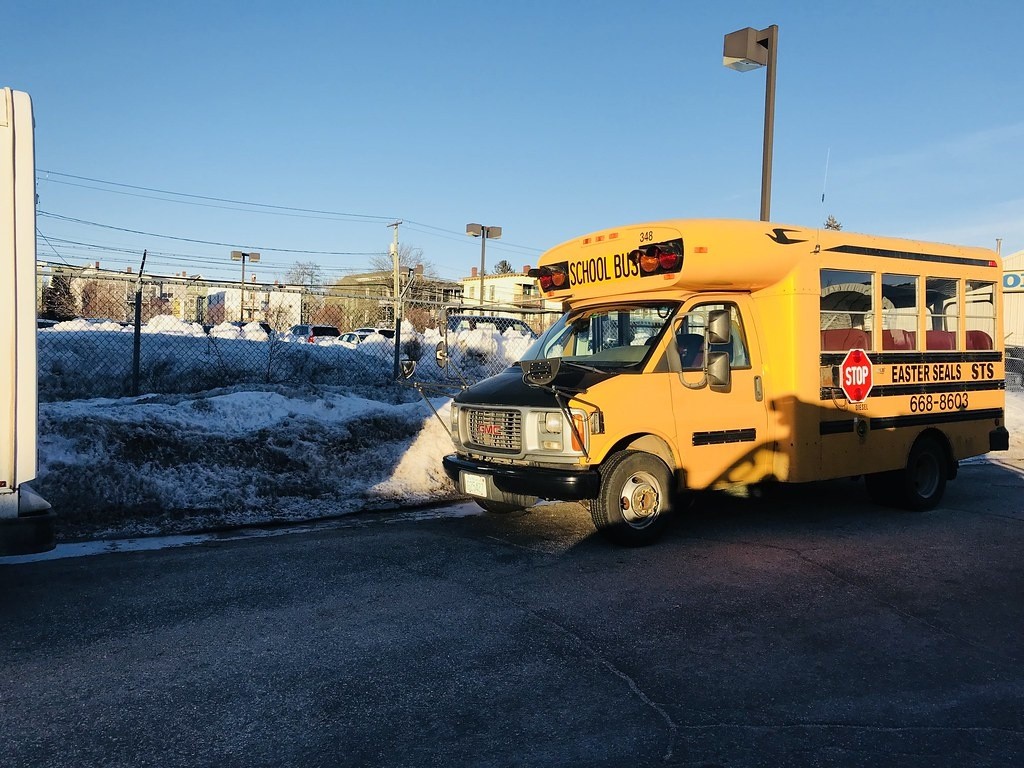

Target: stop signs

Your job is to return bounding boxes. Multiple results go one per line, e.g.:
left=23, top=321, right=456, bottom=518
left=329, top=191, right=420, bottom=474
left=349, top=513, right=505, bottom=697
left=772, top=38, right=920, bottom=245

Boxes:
left=838, top=348, right=873, bottom=403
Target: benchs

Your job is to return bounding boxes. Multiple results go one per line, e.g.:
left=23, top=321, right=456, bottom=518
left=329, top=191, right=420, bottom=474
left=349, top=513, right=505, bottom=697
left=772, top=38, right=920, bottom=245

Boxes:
left=862, top=329, right=915, bottom=351
left=820, top=328, right=873, bottom=352
left=646, top=335, right=706, bottom=368
left=909, top=331, right=957, bottom=350
left=949, top=330, right=993, bottom=350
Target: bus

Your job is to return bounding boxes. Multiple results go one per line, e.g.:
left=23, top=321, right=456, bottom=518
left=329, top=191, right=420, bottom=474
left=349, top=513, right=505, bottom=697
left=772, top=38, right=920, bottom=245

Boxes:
left=395, top=148, right=1011, bottom=549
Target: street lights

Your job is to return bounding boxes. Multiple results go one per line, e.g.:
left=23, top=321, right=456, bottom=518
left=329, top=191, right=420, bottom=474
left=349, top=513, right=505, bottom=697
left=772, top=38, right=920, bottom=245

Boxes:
left=466, top=222, right=502, bottom=317
left=230, top=250, right=260, bottom=323
left=722, top=24, right=779, bottom=222
